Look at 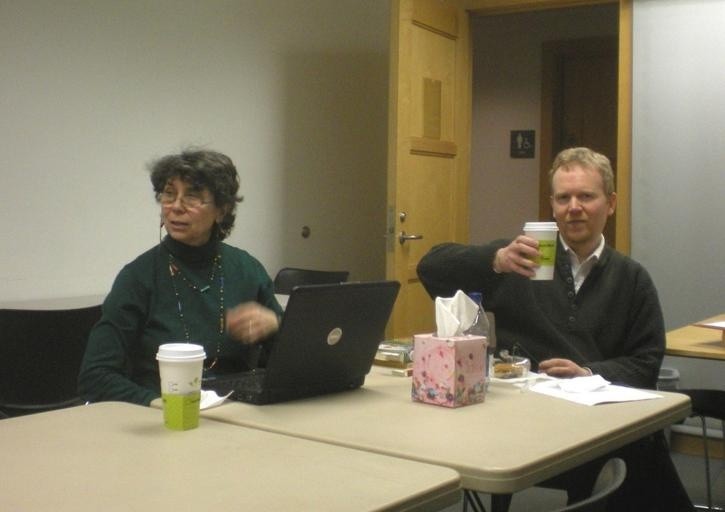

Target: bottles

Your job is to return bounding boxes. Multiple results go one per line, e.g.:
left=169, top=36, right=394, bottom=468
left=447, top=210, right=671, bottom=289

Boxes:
left=467, top=290, right=490, bottom=394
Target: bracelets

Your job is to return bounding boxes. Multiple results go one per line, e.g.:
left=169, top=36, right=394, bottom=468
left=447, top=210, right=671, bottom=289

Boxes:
left=492, top=256, right=502, bottom=274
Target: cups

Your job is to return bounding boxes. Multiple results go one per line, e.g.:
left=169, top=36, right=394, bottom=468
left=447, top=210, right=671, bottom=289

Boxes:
left=524, top=220, right=559, bottom=281
left=156, top=342, right=207, bottom=432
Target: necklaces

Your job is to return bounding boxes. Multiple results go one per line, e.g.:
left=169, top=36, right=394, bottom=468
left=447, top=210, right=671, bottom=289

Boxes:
left=168, top=248, right=229, bottom=371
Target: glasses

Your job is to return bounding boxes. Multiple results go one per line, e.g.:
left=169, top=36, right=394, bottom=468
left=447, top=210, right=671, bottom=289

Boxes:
left=157, top=190, right=218, bottom=208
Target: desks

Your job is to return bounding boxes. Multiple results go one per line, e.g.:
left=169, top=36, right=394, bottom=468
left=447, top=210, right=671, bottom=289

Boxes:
left=665, top=313, right=725, bottom=363
left=0, top=401, right=463, bottom=512
left=148, top=368, right=697, bottom=512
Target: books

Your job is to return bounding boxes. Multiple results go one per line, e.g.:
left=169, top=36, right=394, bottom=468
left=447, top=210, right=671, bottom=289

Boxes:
left=486, top=377, right=544, bottom=388
left=367, top=364, right=414, bottom=377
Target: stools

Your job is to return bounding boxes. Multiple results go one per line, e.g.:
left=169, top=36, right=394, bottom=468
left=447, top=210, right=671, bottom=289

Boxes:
left=656, top=386, right=725, bottom=511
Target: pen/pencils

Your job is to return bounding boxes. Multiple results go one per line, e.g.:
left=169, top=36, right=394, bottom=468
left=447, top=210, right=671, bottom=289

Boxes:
left=515, top=340, right=540, bottom=366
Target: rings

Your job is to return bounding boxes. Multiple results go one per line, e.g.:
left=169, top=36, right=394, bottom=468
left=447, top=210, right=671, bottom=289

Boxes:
left=248, top=321, right=255, bottom=336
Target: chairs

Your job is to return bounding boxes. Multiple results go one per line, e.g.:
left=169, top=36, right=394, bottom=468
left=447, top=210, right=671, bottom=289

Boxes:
left=272, top=268, right=350, bottom=298
left=1, top=301, right=122, bottom=417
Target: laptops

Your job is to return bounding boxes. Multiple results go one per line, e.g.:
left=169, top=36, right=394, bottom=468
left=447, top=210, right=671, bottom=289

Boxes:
left=201, top=280, right=401, bottom=405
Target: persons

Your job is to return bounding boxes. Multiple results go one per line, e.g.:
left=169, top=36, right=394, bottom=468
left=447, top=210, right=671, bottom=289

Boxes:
left=416, top=147, right=666, bottom=390
left=77, top=149, right=288, bottom=409
